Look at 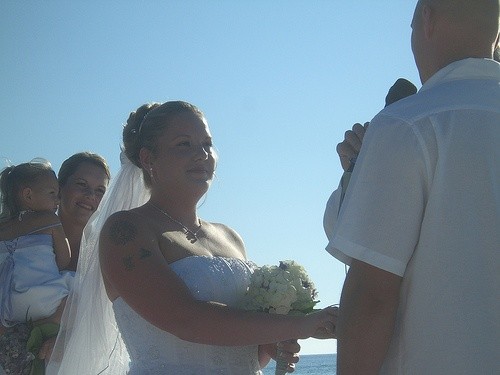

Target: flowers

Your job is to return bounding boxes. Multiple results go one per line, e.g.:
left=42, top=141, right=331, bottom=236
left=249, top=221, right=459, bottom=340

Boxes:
left=0, top=305, right=63, bottom=375
left=239, top=259, right=321, bottom=375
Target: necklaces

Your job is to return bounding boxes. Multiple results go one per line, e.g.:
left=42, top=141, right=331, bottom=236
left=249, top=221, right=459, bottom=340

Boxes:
left=148, top=201, right=202, bottom=240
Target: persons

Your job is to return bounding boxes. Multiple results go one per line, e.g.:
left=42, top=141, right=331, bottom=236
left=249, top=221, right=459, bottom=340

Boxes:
left=322, top=0, right=500, bottom=374
left=46, top=99, right=339, bottom=375
left=0, top=157, right=77, bottom=327
left=0, top=152, right=112, bottom=375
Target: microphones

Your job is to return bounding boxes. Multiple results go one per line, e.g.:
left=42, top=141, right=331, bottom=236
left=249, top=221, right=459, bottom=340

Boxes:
left=340, top=78, right=418, bottom=187
